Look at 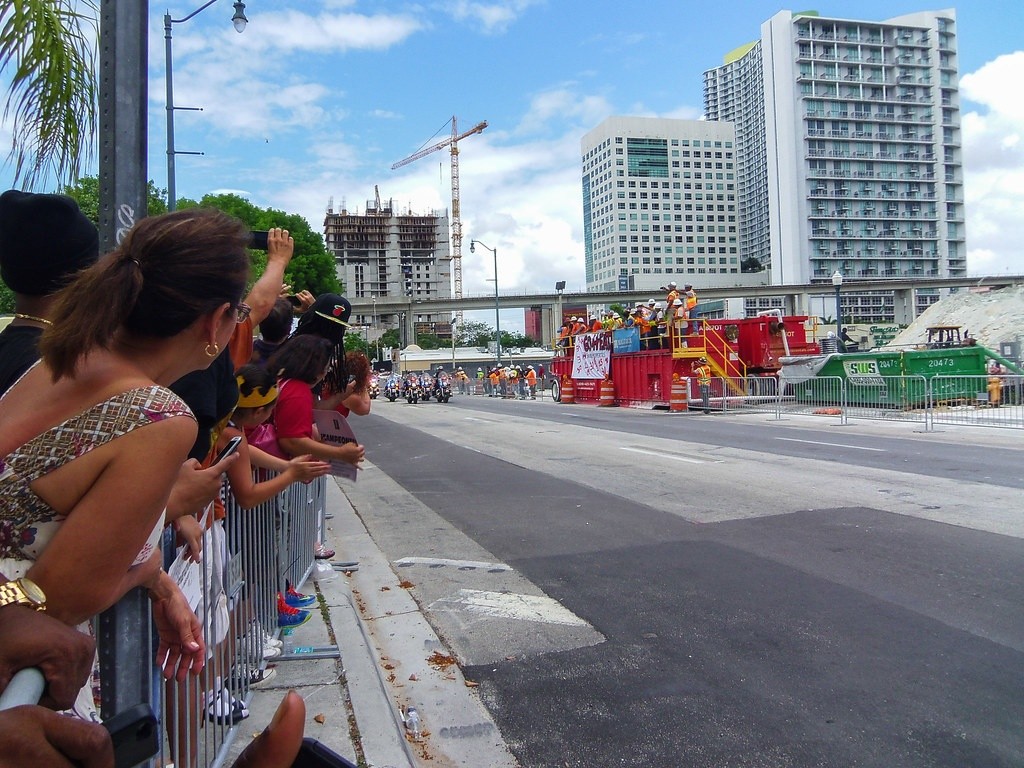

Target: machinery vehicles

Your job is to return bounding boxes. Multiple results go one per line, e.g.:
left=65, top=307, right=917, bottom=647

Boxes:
left=911, top=326, right=977, bottom=350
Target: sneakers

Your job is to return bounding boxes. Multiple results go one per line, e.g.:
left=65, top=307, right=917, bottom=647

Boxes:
left=245, top=624, right=283, bottom=649
left=224, top=661, right=275, bottom=695
left=235, top=636, right=281, bottom=660
left=273, top=592, right=312, bottom=627
left=285, top=586, right=317, bottom=608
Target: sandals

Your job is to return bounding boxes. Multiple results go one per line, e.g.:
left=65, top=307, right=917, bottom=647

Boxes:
left=195, top=694, right=249, bottom=725
left=217, top=687, right=246, bottom=709
left=314, top=543, right=335, bottom=559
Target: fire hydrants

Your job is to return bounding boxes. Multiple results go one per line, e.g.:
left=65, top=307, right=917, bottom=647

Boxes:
left=986, top=377, right=1003, bottom=407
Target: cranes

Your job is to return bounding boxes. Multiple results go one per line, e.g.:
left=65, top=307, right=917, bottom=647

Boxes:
left=326, top=196, right=338, bottom=217
left=340, top=199, right=350, bottom=216
left=384, top=198, right=393, bottom=213
left=390, top=115, right=490, bottom=327
left=366, top=185, right=382, bottom=214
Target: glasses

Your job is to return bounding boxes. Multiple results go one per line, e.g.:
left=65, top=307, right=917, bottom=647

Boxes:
left=210, top=299, right=251, bottom=324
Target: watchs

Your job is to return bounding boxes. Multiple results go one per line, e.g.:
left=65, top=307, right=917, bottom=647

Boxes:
left=0, top=577, right=46, bottom=611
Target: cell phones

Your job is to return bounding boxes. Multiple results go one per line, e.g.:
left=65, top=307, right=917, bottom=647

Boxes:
left=209, top=437, right=242, bottom=468
left=246, top=230, right=269, bottom=250
left=289, top=737, right=359, bottom=768
left=99, top=703, right=159, bottom=768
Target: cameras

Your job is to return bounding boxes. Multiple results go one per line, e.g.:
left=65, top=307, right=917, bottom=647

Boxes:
left=287, top=296, right=302, bottom=309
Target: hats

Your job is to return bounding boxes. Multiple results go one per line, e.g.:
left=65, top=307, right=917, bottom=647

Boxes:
left=0, top=190, right=99, bottom=296
left=315, top=294, right=351, bottom=328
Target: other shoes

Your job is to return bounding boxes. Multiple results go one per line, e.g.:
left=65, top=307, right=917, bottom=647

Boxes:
left=458, top=391, right=536, bottom=401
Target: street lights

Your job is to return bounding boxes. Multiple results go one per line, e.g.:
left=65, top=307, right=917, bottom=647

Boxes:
left=832, top=271, right=843, bottom=353
left=164, top=0, right=248, bottom=213
left=469, top=239, right=502, bottom=368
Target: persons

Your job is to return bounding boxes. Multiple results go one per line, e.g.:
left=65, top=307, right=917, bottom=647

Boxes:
left=554, top=299, right=668, bottom=356
left=660, top=282, right=679, bottom=335
left=0, top=190, right=370, bottom=768
left=455, top=366, right=471, bottom=395
left=675, top=283, right=699, bottom=336
left=475, top=367, right=484, bottom=378
left=538, top=364, right=545, bottom=389
left=483, top=363, right=537, bottom=400
left=372, top=366, right=452, bottom=396
left=841, top=328, right=855, bottom=352
left=691, top=356, right=711, bottom=414
left=671, top=299, right=688, bottom=348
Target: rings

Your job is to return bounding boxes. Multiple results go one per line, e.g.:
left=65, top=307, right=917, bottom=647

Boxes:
left=160, top=567, right=162, bottom=573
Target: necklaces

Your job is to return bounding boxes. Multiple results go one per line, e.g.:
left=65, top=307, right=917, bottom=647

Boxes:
left=14, top=313, right=53, bottom=326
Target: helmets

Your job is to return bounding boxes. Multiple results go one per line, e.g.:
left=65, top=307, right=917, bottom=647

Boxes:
left=370, top=370, right=373, bottom=374
left=699, top=356, right=708, bottom=364
left=437, top=368, right=439, bottom=370
left=408, top=370, right=411, bottom=373
left=438, top=366, right=443, bottom=370
left=458, top=362, right=543, bottom=372
left=563, top=282, right=693, bottom=322
left=421, top=370, right=425, bottom=374
left=390, top=371, right=393, bottom=374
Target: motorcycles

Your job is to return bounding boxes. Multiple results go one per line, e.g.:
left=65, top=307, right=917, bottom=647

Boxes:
left=384, top=377, right=400, bottom=402
left=401, top=373, right=421, bottom=404
left=369, top=379, right=381, bottom=399
left=432, top=371, right=453, bottom=403
left=418, top=373, right=435, bottom=400
